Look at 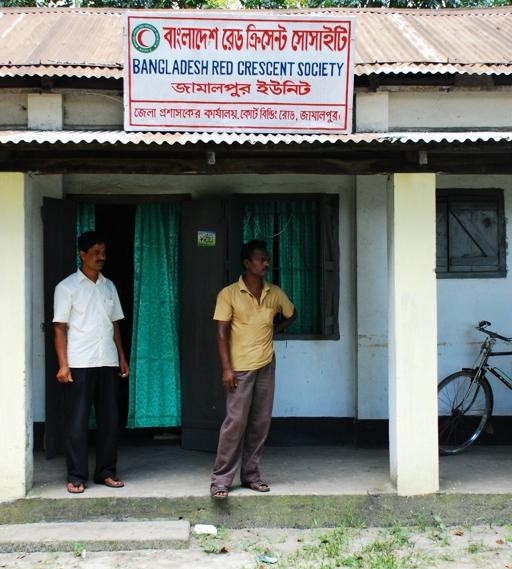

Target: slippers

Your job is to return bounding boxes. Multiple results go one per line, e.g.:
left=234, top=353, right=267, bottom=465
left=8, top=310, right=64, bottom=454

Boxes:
left=210, top=482, right=228, bottom=499
left=66, top=480, right=85, bottom=493
left=94, top=475, right=125, bottom=487
left=249, top=481, right=270, bottom=492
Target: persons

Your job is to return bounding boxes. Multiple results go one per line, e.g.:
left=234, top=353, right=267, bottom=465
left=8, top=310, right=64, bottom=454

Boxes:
left=210, top=240, right=296, bottom=500
left=52, top=230, right=129, bottom=493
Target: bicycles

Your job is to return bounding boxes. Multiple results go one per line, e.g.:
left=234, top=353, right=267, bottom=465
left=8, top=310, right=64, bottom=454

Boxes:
left=438, top=320, right=512, bottom=455
